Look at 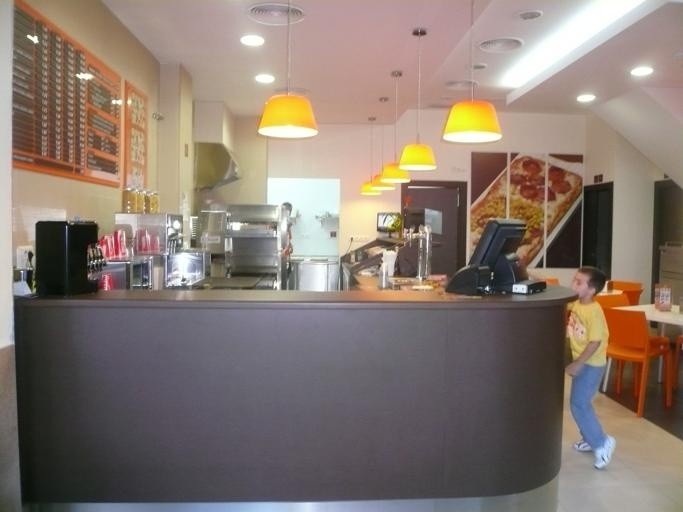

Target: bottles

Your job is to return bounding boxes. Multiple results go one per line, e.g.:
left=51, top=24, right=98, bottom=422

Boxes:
left=655, top=284, right=672, bottom=312
left=123, top=187, right=158, bottom=214
left=376, top=264, right=389, bottom=288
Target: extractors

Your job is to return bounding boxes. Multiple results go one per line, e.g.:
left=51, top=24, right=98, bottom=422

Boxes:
left=193, top=142, right=242, bottom=193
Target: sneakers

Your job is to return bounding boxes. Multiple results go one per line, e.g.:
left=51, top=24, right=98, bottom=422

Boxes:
left=572, top=440, right=594, bottom=451
left=593, top=435, right=616, bottom=469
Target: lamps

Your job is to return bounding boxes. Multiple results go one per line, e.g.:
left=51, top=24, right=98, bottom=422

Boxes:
left=358, top=0, right=502, bottom=196
left=256, top=0, right=320, bottom=140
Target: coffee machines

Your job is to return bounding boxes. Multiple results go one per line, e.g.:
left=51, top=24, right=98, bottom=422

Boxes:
left=37, top=221, right=101, bottom=298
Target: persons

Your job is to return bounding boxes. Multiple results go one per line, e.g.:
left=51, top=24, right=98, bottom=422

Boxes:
left=563, top=266, right=617, bottom=470
left=278, top=202, right=296, bottom=290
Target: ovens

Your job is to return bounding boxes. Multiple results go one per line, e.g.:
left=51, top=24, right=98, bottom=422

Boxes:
left=114, top=212, right=188, bottom=288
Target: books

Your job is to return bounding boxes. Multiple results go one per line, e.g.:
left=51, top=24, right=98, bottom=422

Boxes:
left=411, top=279, right=444, bottom=290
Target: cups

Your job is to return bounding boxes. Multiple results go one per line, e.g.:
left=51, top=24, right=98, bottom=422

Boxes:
left=14, top=268, right=33, bottom=293
left=136, top=228, right=161, bottom=254
left=99, top=231, right=127, bottom=290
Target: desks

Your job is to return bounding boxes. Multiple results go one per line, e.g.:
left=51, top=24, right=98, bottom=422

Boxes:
left=599, top=302, right=683, bottom=395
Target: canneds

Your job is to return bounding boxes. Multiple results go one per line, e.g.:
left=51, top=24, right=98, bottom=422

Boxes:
left=123, top=188, right=160, bottom=214
left=136, top=229, right=160, bottom=252
left=100, top=228, right=126, bottom=291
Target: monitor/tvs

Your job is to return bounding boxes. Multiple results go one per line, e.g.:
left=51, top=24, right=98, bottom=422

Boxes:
left=376, top=213, right=400, bottom=238
left=468, top=217, right=526, bottom=271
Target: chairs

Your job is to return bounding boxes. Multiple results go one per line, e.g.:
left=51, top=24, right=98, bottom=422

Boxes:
left=594, top=293, right=630, bottom=307
left=672, top=335, right=682, bottom=393
left=546, top=278, right=559, bottom=285
left=622, top=289, right=644, bottom=305
left=602, top=305, right=672, bottom=419
